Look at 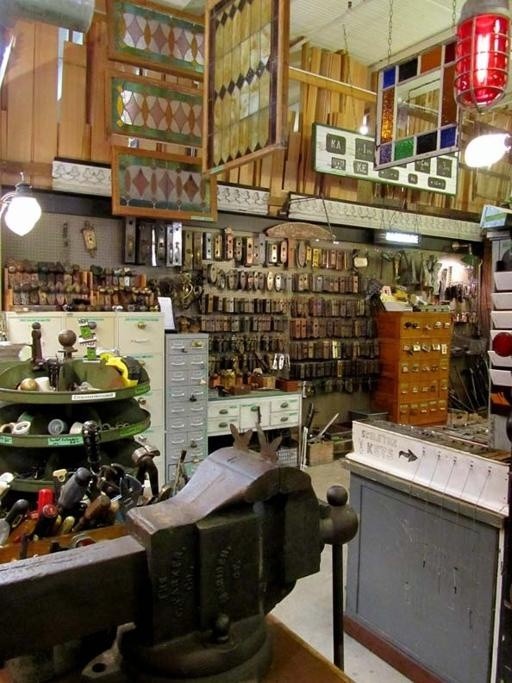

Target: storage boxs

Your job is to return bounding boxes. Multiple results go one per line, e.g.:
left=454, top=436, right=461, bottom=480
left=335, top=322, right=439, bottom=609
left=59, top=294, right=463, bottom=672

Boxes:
left=307, top=441, right=334, bottom=466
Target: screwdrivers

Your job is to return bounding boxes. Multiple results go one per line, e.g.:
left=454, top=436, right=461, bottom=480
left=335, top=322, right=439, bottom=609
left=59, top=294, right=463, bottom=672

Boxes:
left=0, top=467, right=120, bottom=545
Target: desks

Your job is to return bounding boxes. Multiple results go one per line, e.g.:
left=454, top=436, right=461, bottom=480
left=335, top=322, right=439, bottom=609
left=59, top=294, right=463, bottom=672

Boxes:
left=208, top=386, right=305, bottom=472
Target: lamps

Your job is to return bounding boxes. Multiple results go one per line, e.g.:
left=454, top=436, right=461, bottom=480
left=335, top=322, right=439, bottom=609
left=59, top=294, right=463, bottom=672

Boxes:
left=5, top=182, right=43, bottom=236
left=464, top=132, right=512, bottom=167
left=452, top=0, right=512, bottom=116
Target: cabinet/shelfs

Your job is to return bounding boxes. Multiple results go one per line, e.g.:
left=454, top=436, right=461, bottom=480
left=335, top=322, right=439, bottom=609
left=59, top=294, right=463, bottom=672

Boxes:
left=165, top=333, right=209, bottom=485
left=5, top=311, right=165, bottom=495
left=376, top=311, right=453, bottom=426
